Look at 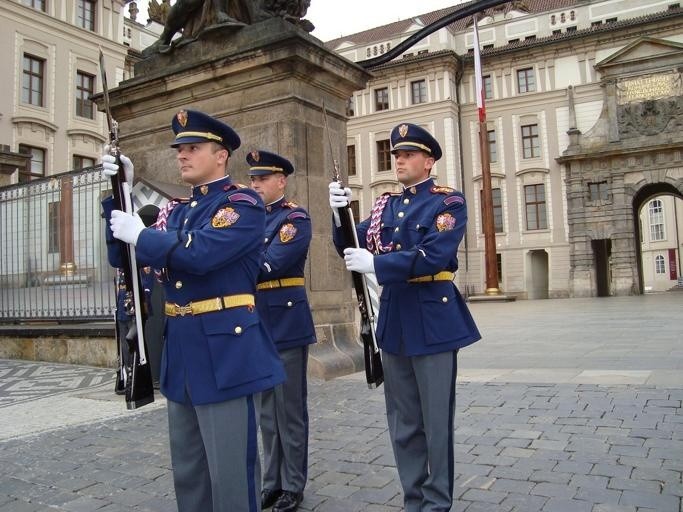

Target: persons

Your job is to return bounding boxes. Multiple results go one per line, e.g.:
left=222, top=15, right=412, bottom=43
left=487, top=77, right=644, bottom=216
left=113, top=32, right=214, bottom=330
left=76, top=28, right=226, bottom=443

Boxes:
left=103, top=109, right=285, bottom=512
left=245, top=151, right=318, bottom=512
left=328, top=123, right=482, bottom=512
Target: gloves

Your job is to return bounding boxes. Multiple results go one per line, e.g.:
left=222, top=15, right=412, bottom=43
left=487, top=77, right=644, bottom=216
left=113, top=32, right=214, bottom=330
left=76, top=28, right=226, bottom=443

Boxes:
left=328, top=181, right=352, bottom=227
left=110, top=209, right=148, bottom=246
left=343, top=248, right=374, bottom=274
left=101, top=144, right=134, bottom=195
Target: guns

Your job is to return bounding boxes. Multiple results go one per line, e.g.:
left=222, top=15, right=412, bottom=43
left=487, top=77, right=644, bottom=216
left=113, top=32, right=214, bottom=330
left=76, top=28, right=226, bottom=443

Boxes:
left=113, top=276, right=126, bottom=395
left=99, top=44, right=154, bottom=410
left=321, top=99, right=383, bottom=389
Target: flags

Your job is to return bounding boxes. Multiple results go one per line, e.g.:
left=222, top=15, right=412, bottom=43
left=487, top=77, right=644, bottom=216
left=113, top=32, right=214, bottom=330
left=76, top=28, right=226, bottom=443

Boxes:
left=474, top=20, right=486, bottom=123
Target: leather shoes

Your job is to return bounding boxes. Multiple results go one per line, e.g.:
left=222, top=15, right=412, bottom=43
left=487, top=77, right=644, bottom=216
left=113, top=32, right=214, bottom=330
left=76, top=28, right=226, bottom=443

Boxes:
left=260, top=486, right=304, bottom=511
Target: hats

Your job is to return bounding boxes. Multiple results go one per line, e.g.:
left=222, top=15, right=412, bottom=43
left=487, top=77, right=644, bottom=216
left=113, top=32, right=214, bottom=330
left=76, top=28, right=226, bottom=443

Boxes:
left=391, top=122, right=442, bottom=161
left=169, top=109, right=241, bottom=156
left=246, top=150, right=294, bottom=177
